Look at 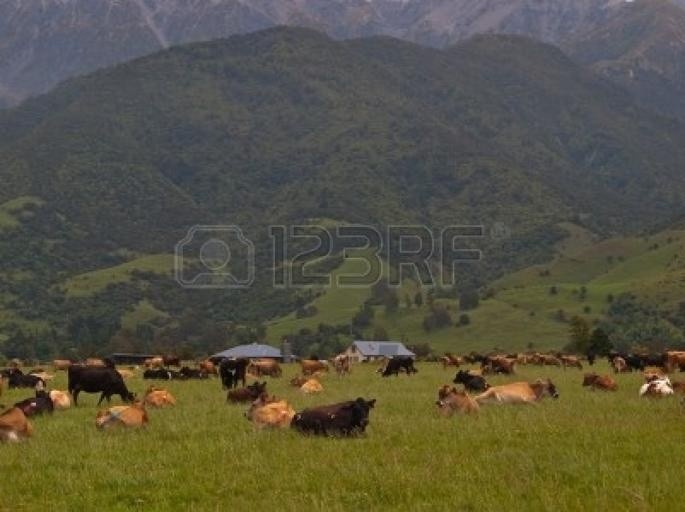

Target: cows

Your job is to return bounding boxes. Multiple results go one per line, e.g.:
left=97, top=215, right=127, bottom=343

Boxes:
left=375, top=353, right=419, bottom=379
left=301, top=357, right=331, bottom=377
left=431, top=351, right=685, bottom=415
left=290, top=396, right=376, bottom=440
left=0, top=349, right=281, bottom=407
left=291, top=376, right=324, bottom=395
left=243, top=390, right=293, bottom=427
left=333, top=352, right=351, bottom=379
left=0, top=404, right=34, bottom=446
left=95, top=400, right=150, bottom=428
